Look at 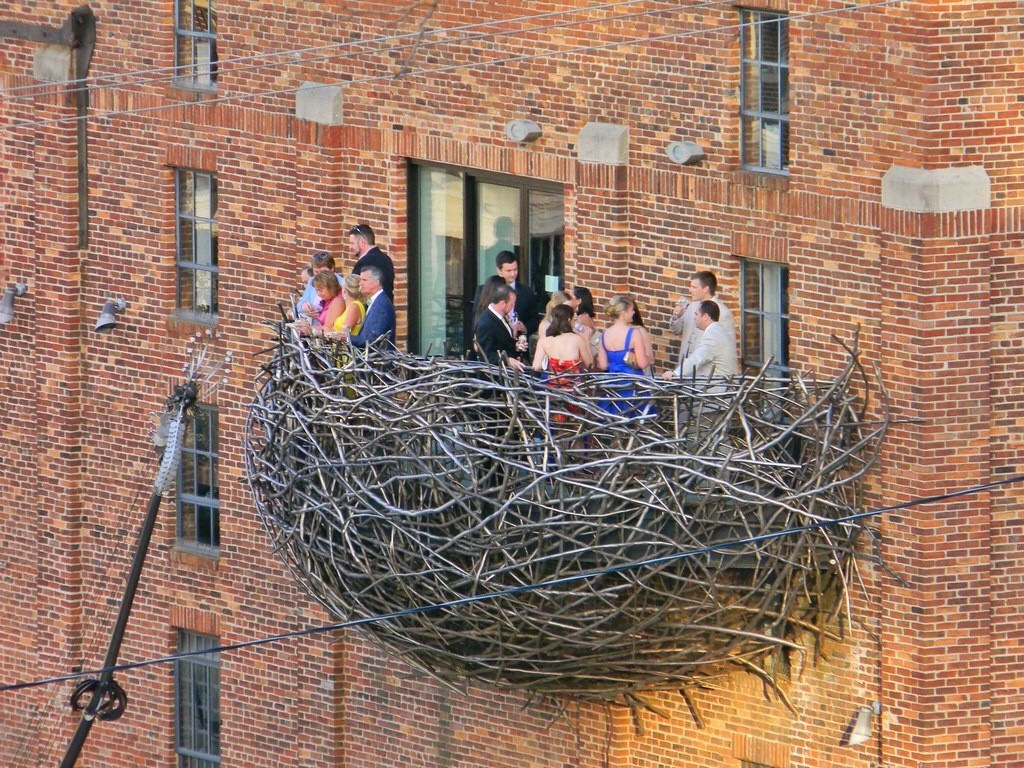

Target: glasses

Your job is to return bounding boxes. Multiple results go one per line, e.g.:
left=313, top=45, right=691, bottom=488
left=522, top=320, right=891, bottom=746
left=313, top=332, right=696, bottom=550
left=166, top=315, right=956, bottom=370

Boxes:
left=312, top=253, right=334, bottom=265
left=351, top=225, right=366, bottom=239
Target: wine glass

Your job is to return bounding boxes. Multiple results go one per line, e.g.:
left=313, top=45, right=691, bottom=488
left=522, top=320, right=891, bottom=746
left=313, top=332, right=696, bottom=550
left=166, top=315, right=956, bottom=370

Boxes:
left=673, top=297, right=690, bottom=320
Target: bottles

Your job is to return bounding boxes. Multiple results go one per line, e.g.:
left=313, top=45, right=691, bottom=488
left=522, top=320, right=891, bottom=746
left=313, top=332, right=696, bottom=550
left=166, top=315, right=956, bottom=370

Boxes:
left=517, top=335, right=527, bottom=350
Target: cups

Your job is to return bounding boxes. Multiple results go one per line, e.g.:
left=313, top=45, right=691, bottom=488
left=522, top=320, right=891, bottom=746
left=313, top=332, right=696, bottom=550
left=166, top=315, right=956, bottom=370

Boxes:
left=510, top=312, right=518, bottom=326
left=340, top=325, right=351, bottom=334
left=573, top=321, right=585, bottom=333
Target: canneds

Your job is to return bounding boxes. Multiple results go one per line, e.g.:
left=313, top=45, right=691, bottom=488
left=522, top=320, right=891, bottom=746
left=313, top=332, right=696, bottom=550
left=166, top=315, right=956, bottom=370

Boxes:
left=517, top=335, right=527, bottom=350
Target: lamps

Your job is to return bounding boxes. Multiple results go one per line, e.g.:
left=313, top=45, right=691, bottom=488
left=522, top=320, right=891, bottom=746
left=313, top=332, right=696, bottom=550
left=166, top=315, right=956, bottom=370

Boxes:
left=662, top=141, right=705, bottom=167
left=0, top=283, right=26, bottom=324
left=502, top=117, right=543, bottom=142
left=849, top=701, right=880, bottom=745
left=95, top=299, right=127, bottom=331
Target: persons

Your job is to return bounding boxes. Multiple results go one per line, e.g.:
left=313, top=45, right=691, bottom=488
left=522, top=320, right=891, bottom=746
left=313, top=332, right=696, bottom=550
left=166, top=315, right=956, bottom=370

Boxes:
left=349, top=224, right=394, bottom=304
left=460, top=274, right=504, bottom=426
left=533, top=287, right=661, bottom=486
left=284, top=251, right=365, bottom=399
left=478, top=284, right=526, bottom=493
left=329, top=266, right=400, bottom=454
left=662, top=271, right=739, bottom=494
left=495, top=249, right=539, bottom=359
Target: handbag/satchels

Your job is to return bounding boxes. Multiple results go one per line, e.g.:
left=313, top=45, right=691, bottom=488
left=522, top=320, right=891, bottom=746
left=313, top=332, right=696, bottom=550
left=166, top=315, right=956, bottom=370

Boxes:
left=624, top=352, right=639, bottom=367
